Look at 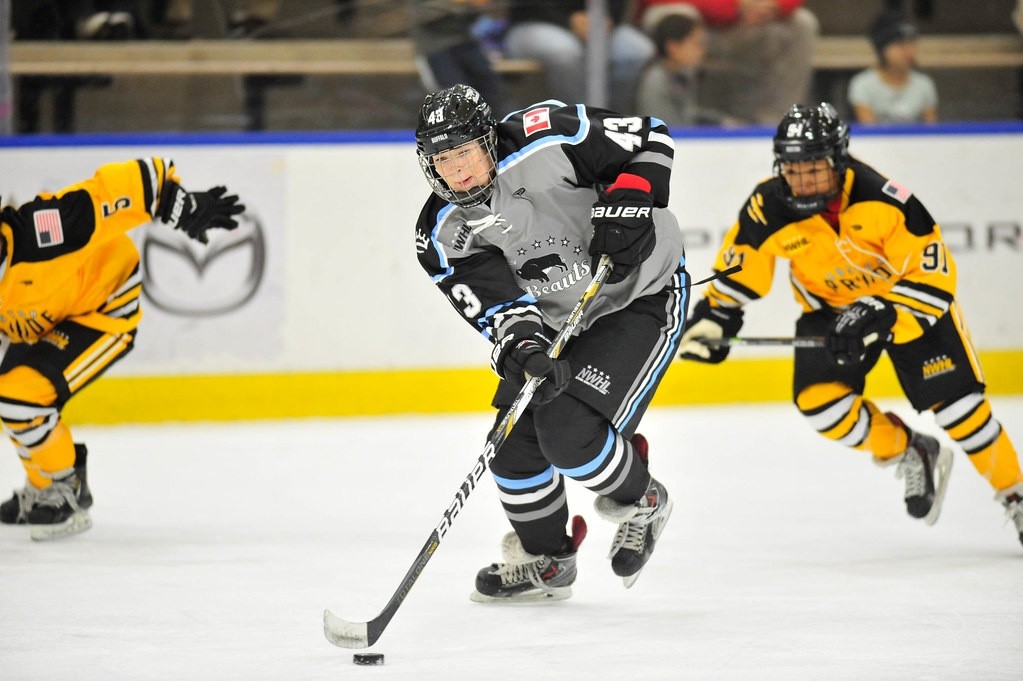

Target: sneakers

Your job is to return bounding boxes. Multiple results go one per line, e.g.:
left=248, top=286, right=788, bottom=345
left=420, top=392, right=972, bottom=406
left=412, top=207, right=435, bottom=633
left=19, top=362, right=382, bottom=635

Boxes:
left=0, top=483, right=40, bottom=538
left=31, top=444, right=92, bottom=543
left=594, top=433, right=674, bottom=588
left=471, top=516, right=587, bottom=604
left=991, top=480, right=1023, bottom=547
left=873, top=411, right=954, bottom=526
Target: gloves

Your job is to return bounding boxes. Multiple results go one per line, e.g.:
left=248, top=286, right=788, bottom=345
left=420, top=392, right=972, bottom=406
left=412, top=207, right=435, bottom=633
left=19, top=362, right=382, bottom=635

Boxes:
left=588, top=188, right=656, bottom=283
left=679, top=299, right=745, bottom=363
left=159, top=179, right=245, bottom=244
left=826, top=294, right=897, bottom=378
left=490, top=330, right=572, bottom=408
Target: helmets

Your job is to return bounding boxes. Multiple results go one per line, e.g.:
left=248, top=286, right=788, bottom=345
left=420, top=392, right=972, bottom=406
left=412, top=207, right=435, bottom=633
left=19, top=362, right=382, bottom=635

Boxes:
left=415, top=84, right=499, bottom=208
left=772, top=103, right=850, bottom=212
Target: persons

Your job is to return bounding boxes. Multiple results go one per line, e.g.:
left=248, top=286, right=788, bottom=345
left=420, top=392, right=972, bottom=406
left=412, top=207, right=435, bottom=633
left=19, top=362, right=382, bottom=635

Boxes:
left=848, top=12, right=939, bottom=126
left=8, top=0, right=82, bottom=136
left=409, top=0, right=491, bottom=94
left=677, top=101, right=1023, bottom=548
left=502, top=0, right=655, bottom=106
left=414, top=84, right=743, bottom=605
left=0, top=154, right=246, bottom=541
left=641, top=0, right=817, bottom=128
left=635, top=12, right=750, bottom=130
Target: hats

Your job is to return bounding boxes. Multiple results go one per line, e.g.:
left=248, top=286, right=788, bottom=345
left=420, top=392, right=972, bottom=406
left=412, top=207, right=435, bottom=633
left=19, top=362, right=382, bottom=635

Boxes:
left=870, top=11, right=918, bottom=44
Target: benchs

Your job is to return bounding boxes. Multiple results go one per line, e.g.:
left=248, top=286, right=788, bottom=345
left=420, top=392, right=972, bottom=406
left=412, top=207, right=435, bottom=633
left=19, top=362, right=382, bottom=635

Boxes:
left=10, top=33, right=1023, bottom=135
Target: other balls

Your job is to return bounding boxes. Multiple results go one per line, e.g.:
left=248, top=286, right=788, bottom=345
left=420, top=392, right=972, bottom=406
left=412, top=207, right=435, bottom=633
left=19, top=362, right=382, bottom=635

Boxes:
left=352, top=654, right=385, bottom=666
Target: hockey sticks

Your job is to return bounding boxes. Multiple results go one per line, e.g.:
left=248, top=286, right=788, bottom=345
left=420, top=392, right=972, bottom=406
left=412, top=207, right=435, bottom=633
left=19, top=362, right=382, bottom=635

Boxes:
left=323, top=255, right=611, bottom=651
left=678, top=337, right=824, bottom=347
